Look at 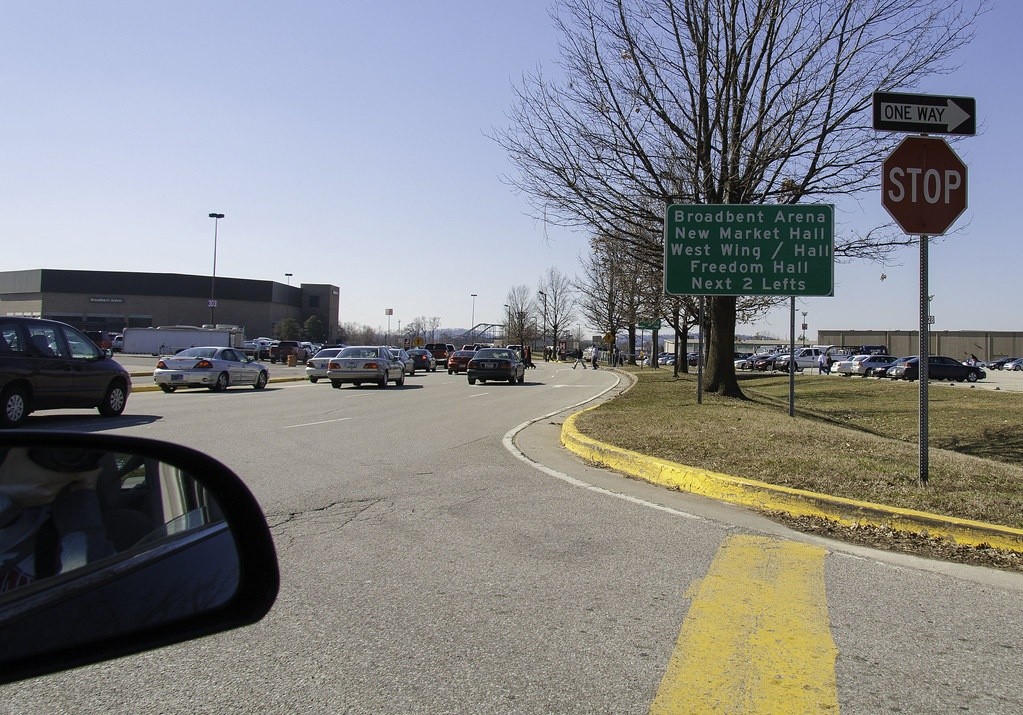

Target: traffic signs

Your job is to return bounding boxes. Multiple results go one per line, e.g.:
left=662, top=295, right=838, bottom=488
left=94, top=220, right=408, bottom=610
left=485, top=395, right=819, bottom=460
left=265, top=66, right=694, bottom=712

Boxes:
left=873, top=91, right=976, bottom=136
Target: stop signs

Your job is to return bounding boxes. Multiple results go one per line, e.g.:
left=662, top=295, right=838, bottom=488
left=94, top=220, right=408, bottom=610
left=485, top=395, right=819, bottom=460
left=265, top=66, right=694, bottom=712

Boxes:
left=882, top=135, right=968, bottom=236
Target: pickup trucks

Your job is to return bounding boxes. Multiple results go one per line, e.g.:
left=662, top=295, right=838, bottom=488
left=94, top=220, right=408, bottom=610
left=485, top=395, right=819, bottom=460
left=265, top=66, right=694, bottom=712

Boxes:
left=269, top=340, right=308, bottom=364
left=775, top=348, right=847, bottom=373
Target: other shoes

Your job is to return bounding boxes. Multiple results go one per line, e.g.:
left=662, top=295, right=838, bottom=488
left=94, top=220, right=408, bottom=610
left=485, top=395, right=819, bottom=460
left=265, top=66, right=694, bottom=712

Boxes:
left=597, top=365, right=599, bottom=367
left=572, top=366, right=575, bottom=369
left=621, top=363, right=624, bottom=367
left=583, top=367, right=587, bottom=369
left=593, top=368, right=596, bottom=370
left=613, top=365, right=616, bottom=367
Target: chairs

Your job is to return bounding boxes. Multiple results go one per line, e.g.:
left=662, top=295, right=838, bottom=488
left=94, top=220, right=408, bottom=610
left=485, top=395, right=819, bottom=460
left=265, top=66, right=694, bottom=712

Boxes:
left=32, top=335, right=58, bottom=355
left=101, top=453, right=155, bottom=546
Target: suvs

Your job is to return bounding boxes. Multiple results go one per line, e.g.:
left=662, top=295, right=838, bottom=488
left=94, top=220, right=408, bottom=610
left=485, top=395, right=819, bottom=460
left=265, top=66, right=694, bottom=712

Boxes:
left=0, top=317, right=132, bottom=428
left=567, top=346, right=610, bottom=362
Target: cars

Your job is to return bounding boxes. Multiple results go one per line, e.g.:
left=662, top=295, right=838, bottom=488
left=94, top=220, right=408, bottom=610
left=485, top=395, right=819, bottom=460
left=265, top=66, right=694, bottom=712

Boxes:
left=831, top=355, right=987, bottom=382
left=734, top=353, right=804, bottom=372
left=372, top=344, right=523, bottom=375
left=153, top=346, right=270, bottom=393
left=327, top=346, right=406, bottom=388
left=243, top=336, right=346, bottom=383
left=962, top=357, right=1023, bottom=372
left=467, top=348, right=526, bottom=386
left=646, top=352, right=704, bottom=366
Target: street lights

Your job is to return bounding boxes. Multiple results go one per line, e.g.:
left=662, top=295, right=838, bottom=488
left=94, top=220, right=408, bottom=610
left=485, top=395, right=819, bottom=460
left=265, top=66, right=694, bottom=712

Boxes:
left=208, top=212, right=224, bottom=324
left=471, top=294, right=477, bottom=344
left=505, top=304, right=510, bottom=345
left=927, top=294, right=935, bottom=355
left=802, top=311, right=808, bottom=347
left=539, top=290, right=546, bottom=346
left=576, top=323, right=581, bottom=348
left=285, top=272, right=293, bottom=317
left=534, top=316, right=537, bottom=351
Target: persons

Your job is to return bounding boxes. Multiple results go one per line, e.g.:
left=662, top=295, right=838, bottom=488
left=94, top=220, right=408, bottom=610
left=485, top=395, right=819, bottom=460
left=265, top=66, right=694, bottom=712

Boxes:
left=544, top=347, right=563, bottom=362
left=826, top=352, right=833, bottom=375
left=817, top=351, right=827, bottom=375
left=613, top=344, right=623, bottom=367
left=515, top=346, right=536, bottom=369
left=572, top=347, right=587, bottom=369
left=965, top=351, right=978, bottom=367
left=592, top=344, right=599, bottom=370
left=474, top=344, right=499, bottom=351
left=0, top=445, right=154, bottom=591
